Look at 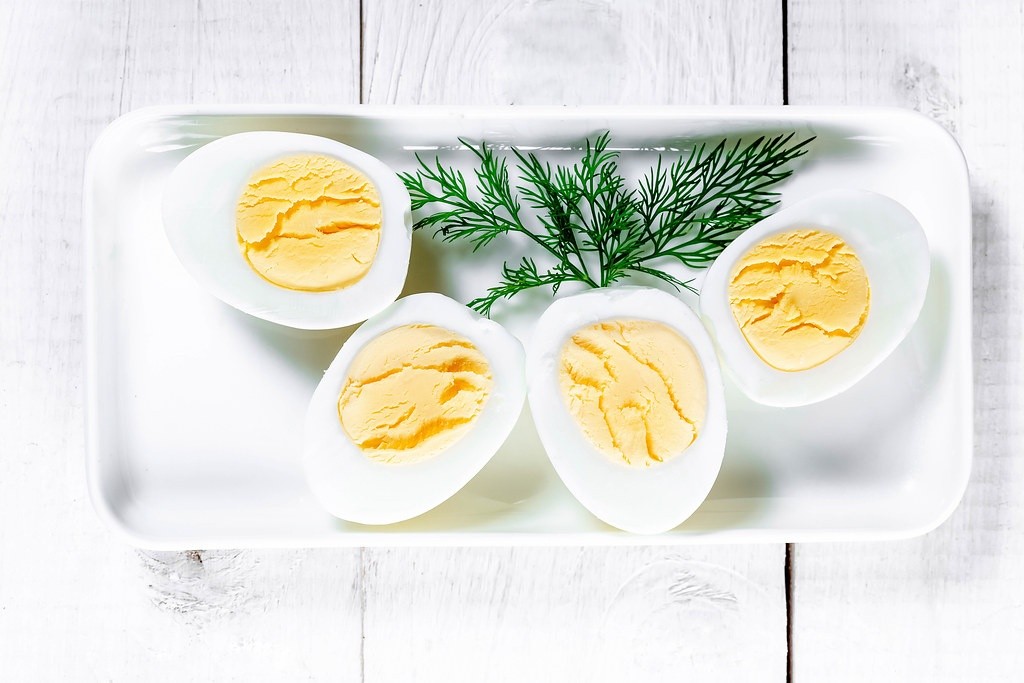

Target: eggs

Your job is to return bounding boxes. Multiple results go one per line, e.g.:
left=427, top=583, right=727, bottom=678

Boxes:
left=164, top=130, right=932, bottom=536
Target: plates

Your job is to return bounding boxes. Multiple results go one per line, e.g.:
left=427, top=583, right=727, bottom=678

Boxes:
left=80, top=105, right=982, bottom=550
